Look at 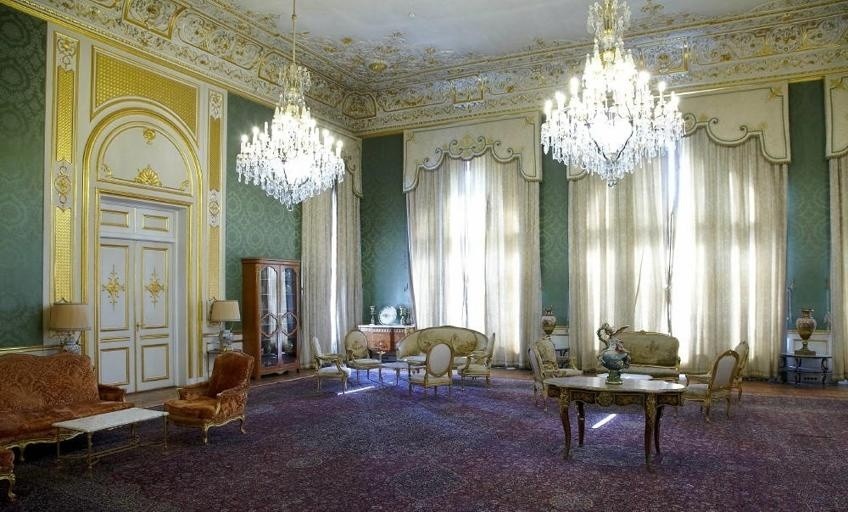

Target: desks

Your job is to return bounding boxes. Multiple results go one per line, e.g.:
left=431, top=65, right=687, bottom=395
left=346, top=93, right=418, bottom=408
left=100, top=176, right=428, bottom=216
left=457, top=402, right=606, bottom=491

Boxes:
left=542, top=372, right=688, bottom=461
left=580, top=365, right=676, bottom=378
left=371, top=360, right=425, bottom=387
left=51, top=406, right=173, bottom=477
left=774, top=351, right=834, bottom=388
left=207, top=346, right=242, bottom=379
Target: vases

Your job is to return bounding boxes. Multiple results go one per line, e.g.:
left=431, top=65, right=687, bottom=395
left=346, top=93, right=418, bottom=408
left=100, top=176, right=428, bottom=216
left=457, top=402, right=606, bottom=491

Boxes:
left=540, top=305, right=556, bottom=337
left=793, top=308, right=817, bottom=357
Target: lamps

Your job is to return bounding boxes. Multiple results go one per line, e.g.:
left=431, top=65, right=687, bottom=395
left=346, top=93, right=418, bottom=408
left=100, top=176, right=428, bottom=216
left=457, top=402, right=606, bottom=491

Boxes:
left=236, top=0, right=345, bottom=211
left=207, top=300, right=243, bottom=351
left=535, top=1, right=687, bottom=187
left=48, top=299, right=91, bottom=352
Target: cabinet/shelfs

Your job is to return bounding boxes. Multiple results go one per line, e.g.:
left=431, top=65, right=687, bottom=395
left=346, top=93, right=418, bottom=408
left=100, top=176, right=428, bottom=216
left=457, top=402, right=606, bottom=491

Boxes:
left=356, top=323, right=417, bottom=358
left=238, top=255, right=306, bottom=381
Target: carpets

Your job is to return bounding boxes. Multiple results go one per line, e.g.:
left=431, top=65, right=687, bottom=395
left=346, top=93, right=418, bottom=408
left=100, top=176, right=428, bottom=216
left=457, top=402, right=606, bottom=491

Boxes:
left=2, top=368, right=847, bottom=512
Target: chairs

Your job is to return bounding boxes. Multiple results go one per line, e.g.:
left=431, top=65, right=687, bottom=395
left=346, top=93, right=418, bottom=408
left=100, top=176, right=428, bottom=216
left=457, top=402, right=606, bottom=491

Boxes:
left=672, top=349, right=740, bottom=424
left=532, top=333, right=582, bottom=379
left=344, top=327, right=384, bottom=384
left=457, top=332, right=496, bottom=392
left=684, top=341, right=751, bottom=412
left=406, top=343, right=456, bottom=404
left=528, top=333, right=567, bottom=411
left=309, top=335, right=349, bottom=393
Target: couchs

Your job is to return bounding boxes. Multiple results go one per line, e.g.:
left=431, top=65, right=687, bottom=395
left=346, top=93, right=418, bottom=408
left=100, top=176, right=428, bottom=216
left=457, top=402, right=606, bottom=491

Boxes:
left=166, top=351, right=258, bottom=444
left=595, top=328, right=683, bottom=379
left=392, top=323, right=491, bottom=389
left=1, top=344, right=144, bottom=502
